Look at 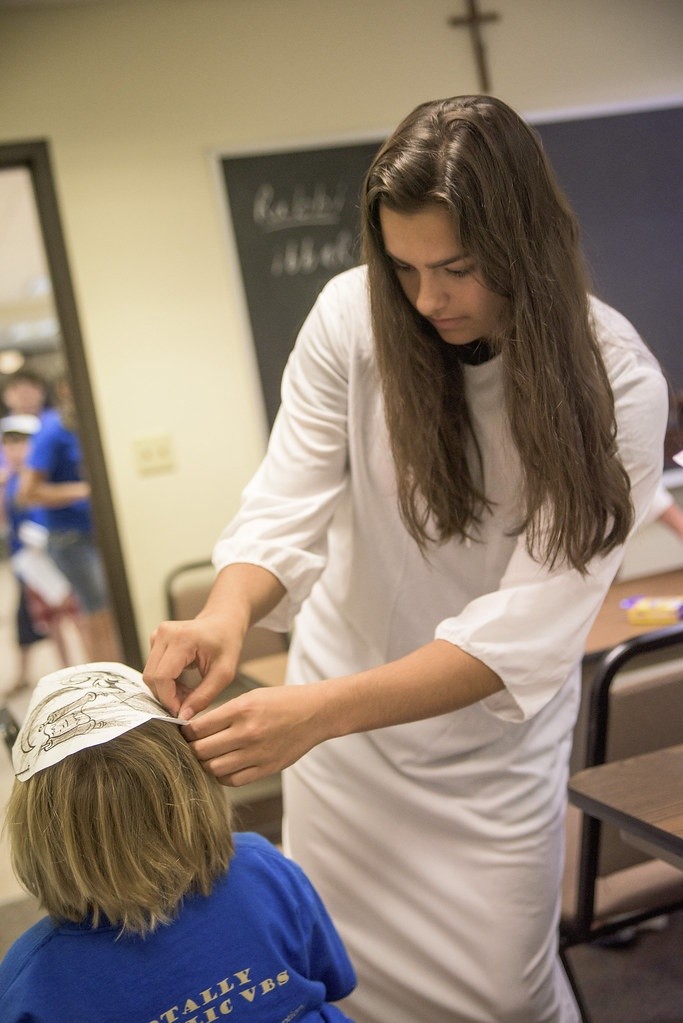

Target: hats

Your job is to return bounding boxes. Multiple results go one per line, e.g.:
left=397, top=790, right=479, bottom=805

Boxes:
left=1, top=414, right=41, bottom=438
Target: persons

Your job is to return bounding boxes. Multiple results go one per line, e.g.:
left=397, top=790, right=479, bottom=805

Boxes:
left=0, top=662, right=359, bottom=1023
left=0, top=349, right=130, bottom=704
left=142, top=95, right=683, bottom=1023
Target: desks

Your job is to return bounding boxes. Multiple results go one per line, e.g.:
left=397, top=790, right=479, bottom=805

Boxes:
left=567, top=743, right=683, bottom=870
left=238, top=567, right=683, bottom=688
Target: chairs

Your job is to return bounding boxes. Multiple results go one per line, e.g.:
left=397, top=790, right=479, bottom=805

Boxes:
left=163, top=560, right=283, bottom=831
left=560, top=626, right=683, bottom=944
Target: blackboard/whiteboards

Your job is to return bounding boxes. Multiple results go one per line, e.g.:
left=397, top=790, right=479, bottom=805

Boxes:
left=223, top=98, right=683, bottom=470
